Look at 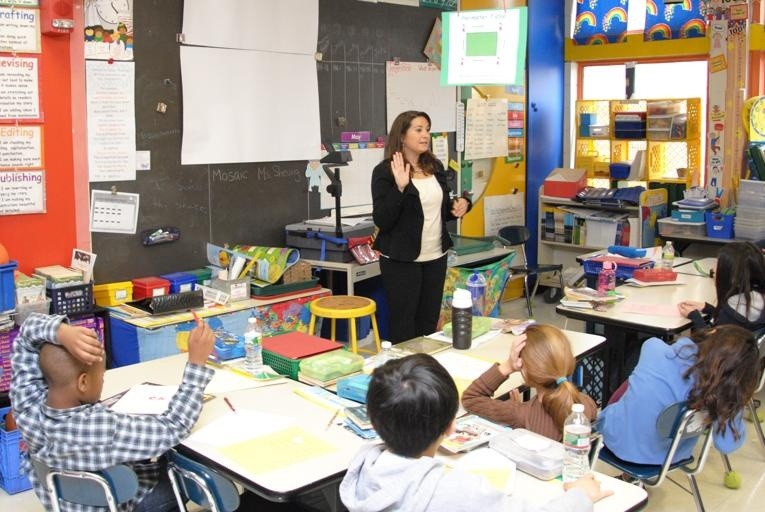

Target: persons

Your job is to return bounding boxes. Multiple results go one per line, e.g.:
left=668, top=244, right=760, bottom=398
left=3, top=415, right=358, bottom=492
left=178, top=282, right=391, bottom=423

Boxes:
left=461, top=323, right=597, bottom=441
left=369, top=110, right=472, bottom=345
left=339, top=353, right=614, bottom=512
left=7, top=312, right=218, bottom=512
left=609, top=243, right=765, bottom=391
left=594, top=325, right=761, bottom=511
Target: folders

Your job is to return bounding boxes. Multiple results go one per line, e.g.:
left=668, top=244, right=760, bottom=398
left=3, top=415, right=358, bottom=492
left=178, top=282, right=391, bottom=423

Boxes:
left=672, top=210, right=705, bottom=222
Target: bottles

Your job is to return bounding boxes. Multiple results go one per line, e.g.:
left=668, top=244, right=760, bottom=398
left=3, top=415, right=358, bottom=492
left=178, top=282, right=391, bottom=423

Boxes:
left=562, top=403, right=593, bottom=483
left=600, top=261, right=617, bottom=296
left=450, top=287, right=473, bottom=349
left=465, top=272, right=488, bottom=324
left=243, top=317, right=264, bottom=376
left=662, top=239, right=675, bottom=272
left=375, top=341, right=396, bottom=367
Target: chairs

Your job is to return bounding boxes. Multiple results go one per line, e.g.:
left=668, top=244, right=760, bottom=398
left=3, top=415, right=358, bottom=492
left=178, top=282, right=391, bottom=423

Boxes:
left=596, top=394, right=721, bottom=512
left=494, top=223, right=566, bottom=320
left=558, top=415, right=604, bottom=474
left=718, top=327, right=765, bottom=473
left=25, top=455, right=142, bottom=512
left=160, top=444, right=242, bottom=512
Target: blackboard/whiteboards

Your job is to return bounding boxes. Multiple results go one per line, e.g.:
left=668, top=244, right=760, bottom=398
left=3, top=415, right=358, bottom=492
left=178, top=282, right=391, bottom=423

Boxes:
left=90, top=3, right=454, bottom=284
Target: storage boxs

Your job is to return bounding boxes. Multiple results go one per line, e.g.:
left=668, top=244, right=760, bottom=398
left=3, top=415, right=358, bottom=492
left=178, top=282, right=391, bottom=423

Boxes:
left=284, top=218, right=378, bottom=263
left=185, top=265, right=212, bottom=286
left=210, top=270, right=253, bottom=304
left=580, top=98, right=686, bottom=143
left=0, top=252, right=20, bottom=315
left=0, top=405, right=39, bottom=496
left=162, top=271, right=197, bottom=295
left=656, top=176, right=764, bottom=242
left=132, top=272, right=170, bottom=300
left=93, top=280, right=134, bottom=308
left=17, top=297, right=54, bottom=320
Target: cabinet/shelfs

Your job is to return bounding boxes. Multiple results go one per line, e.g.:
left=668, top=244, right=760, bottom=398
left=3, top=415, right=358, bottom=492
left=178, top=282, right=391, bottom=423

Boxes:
left=533, top=183, right=670, bottom=304
left=572, top=95, right=702, bottom=195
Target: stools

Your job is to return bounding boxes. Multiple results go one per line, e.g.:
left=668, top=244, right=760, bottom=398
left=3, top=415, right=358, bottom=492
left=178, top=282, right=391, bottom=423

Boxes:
left=302, top=294, right=386, bottom=361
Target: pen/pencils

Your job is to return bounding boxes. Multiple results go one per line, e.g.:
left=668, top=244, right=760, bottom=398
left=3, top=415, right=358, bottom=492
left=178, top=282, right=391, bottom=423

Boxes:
left=672, top=259, right=696, bottom=268
left=190, top=310, right=220, bottom=364
left=590, top=436, right=602, bottom=473
left=327, top=409, right=339, bottom=429
left=224, top=397, right=236, bottom=411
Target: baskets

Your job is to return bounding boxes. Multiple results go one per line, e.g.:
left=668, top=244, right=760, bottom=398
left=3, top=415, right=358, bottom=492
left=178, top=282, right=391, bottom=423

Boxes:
left=262, top=330, right=348, bottom=381
left=583, top=253, right=656, bottom=279
left=46, top=279, right=94, bottom=316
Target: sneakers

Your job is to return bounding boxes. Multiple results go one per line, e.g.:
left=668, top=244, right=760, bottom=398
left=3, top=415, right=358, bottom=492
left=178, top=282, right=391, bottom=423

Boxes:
left=614, top=475, right=647, bottom=489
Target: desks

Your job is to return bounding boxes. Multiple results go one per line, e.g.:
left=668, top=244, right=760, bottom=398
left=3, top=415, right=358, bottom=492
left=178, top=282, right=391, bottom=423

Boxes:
left=575, top=238, right=693, bottom=280
left=554, top=255, right=721, bottom=406
left=658, top=233, right=765, bottom=261
left=92, top=347, right=388, bottom=512
left=286, top=235, right=513, bottom=320
left=292, top=314, right=609, bottom=430
left=411, top=413, right=650, bottom=512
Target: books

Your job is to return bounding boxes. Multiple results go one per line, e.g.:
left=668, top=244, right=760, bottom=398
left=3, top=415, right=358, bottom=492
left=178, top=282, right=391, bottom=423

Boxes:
left=546, top=206, right=642, bottom=249
left=101, top=380, right=172, bottom=416
left=299, top=350, right=504, bottom=460
left=561, top=254, right=686, bottom=309
left=672, top=197, right=718, bottom=211
left=745, top=144, right=764, bottom=181
left=0, top=248, right=96, bottom=328
